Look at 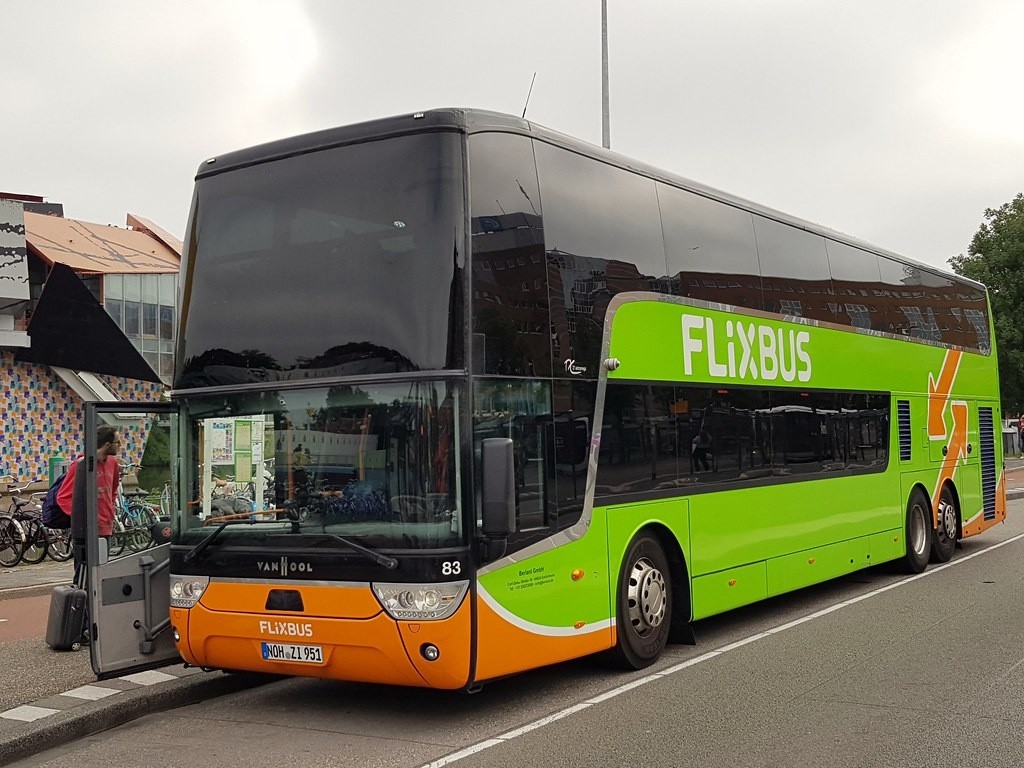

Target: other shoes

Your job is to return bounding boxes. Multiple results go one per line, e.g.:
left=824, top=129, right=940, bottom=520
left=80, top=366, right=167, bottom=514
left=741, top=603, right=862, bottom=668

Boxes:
left=80, top=629, right=90, bottom=646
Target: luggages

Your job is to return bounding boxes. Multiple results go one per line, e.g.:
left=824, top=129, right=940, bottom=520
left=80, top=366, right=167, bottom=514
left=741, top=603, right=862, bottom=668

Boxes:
left=45, top=561, right=88, bottom=652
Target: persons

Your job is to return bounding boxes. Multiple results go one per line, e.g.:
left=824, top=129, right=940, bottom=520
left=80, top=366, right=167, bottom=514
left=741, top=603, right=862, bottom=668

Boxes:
left=1018, top=415, right=1024, bottom=459
left=293, top=443, right=311, bottom=464
left=57, top=425, right=122, bottom=646
left=693, top=430, right=712, bottom=471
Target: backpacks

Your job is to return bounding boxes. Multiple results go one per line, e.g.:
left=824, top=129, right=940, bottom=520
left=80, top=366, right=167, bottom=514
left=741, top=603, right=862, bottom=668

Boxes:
left=42, top=472, right=72, bottom=530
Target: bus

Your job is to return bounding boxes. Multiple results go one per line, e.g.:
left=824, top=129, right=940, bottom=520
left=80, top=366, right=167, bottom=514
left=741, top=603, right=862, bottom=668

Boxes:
left=82, top=108, right=1007, bottom=690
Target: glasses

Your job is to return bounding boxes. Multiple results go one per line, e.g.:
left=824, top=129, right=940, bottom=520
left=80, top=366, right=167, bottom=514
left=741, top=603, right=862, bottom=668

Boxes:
left=103, top=438, right=122, bottom=447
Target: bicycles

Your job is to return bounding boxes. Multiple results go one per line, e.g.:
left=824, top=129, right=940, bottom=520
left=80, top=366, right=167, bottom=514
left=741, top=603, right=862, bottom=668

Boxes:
left=210, top=470, right=392, bottom=520
left=0, top=475, right=172, bottom=567
